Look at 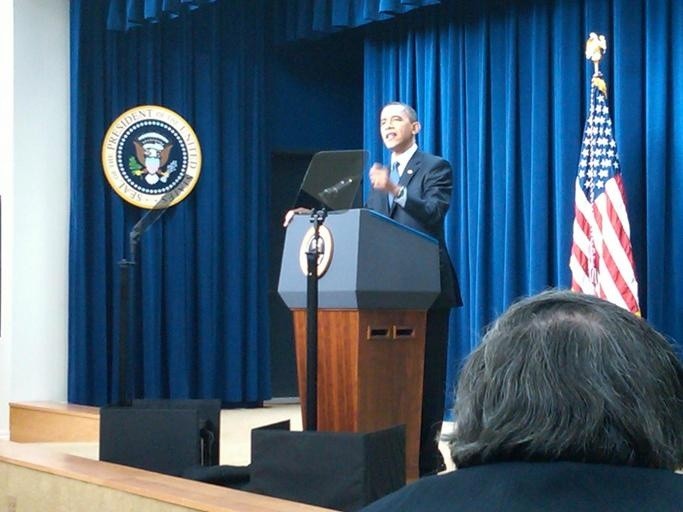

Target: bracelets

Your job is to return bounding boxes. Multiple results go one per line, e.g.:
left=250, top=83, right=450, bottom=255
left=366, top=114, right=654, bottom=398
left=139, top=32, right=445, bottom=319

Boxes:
left=394, top=186, right=405, bottom=198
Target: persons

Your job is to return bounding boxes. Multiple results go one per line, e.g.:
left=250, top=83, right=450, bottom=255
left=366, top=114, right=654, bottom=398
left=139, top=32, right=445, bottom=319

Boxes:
left=280, top=102, right=465, bottom=480
left=365, top=286, right=683, bottom=512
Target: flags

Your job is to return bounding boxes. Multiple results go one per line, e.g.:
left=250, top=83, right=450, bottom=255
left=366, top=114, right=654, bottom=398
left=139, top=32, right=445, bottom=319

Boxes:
left=566, top=28, right=646, bottom=322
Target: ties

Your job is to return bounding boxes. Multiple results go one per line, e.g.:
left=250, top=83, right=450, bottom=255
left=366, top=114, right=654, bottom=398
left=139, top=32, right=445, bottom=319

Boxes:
left=388, top=161, right=400, bottom=208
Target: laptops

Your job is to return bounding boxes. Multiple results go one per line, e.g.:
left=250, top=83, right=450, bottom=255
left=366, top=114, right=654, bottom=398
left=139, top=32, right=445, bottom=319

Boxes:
left=296, top=150, right=368, bottom=211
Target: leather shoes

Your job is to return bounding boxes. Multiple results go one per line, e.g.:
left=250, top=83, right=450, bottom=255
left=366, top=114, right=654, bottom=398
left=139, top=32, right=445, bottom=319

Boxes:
left=426, top=460, right=445, bottom=476
